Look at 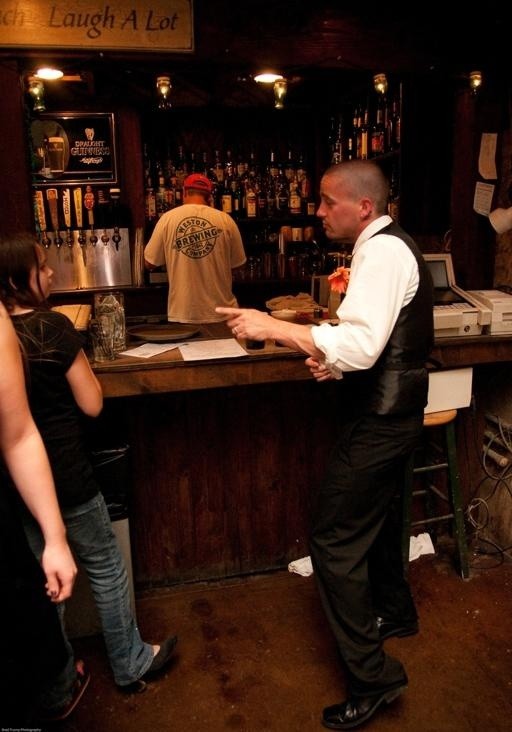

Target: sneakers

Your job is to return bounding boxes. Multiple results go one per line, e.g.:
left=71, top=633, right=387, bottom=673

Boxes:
left=50, top=659, right=90, bottom=720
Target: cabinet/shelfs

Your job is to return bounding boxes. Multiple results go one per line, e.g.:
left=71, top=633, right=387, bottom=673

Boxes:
left=146, top=211, right=332, bottom=313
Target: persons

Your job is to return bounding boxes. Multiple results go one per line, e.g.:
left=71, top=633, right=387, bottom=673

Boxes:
left=1, top=238, right=181, bottom=693
left=0, top=301, right=91, bottom=726
left=141, top=172, right=248, bottom=335
left=216, top=157, right=431, bottom=727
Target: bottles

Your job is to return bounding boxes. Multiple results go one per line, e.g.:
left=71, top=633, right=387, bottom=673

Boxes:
left=142, top=143, right=311, bottom=219
left=382, top=173, right=399, bottom=224
left=340, top=96, right=403, bottom=162
left=230, top=225, right=325, bottom=284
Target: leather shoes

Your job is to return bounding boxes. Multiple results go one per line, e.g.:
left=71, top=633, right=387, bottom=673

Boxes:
left=323, top=667, right=410, bottom=728
left=374, top=592, right=419, bottom=638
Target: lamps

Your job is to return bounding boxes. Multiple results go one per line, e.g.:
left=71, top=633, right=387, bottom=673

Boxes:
left=25, top=76, right=46, bottom=112
left=274, top=79, right=288, bottom=110
left=154, top=77, right=174, bottom=109
left=469, top=70, right=482, bottom=94
left=373, top=72, right=389, bottom=96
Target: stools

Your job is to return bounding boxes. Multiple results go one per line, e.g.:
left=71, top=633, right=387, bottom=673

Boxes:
left=400, top=408, right=473, bottom=583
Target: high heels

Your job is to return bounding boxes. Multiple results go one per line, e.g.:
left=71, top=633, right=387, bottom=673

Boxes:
left=119, top=635, right=178, bottom=692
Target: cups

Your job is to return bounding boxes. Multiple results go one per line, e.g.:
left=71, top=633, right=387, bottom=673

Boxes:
left=292, top=228, right=303, bottom=242
left=88, top=312, right=116, bottom=364
left=94, top=291, right=127, bottom=353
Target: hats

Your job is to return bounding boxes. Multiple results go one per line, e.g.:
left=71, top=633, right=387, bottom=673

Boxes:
left=184, top=174, right=212, bottom=192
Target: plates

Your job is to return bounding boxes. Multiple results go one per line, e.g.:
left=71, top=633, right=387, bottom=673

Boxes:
left=125, top=322, right=204, bottom=341
